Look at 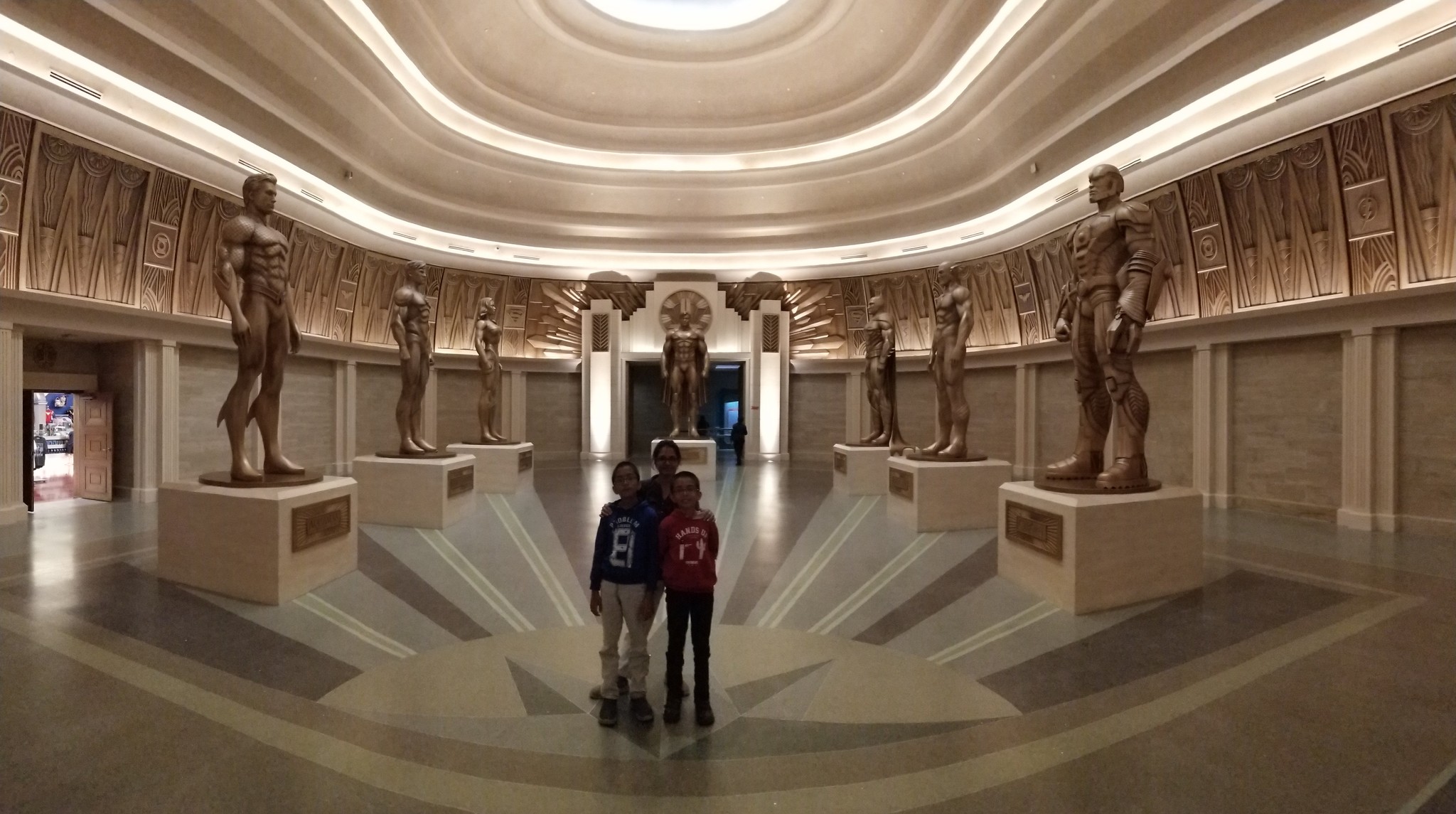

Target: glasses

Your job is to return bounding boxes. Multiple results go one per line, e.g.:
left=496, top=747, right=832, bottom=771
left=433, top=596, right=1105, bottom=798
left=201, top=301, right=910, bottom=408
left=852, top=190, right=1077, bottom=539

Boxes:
left=613, top=476, right=638, bottom=486
left=655, top=456, right=678, bottom=462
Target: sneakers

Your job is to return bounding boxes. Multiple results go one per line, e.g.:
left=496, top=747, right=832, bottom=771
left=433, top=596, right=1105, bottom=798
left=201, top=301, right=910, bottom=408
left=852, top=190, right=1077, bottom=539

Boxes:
left=598, top=698, right=618, bottom=725
left=629, top=695, right=654, bottom=721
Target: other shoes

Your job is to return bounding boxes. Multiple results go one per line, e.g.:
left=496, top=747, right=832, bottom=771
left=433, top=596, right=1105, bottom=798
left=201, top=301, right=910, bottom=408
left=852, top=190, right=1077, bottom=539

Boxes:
left=665, top=670, right=690, bottom=697
left=695, top=698, right=714, bottom=726
left=589, top=676, right=629, bottom=699
left=663, top=691, right=682, bottom=725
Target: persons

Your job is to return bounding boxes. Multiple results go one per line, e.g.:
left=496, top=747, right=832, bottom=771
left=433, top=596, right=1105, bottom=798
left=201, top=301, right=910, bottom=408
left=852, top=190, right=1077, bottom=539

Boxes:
left=730, top=416, right=748, bottom=466
left=215, top=172, right=306, bottom=481
left=660, top=311, right=711, bottom=439
left=696, top=415, right=709, bottom=436
left=589, top=439, right=719, bottom=726
left=390, top=260, right=437, bottom=455
left=51, top=410, right=57, bottom=419
left=922, top=260, right=974, bottom=461
left=65, top=423, right=74, bottom=456
left=1044, top=164, right=1163, bottom=488
left=860, top=296, right=896, bottom=446
left=475, top=297, right=507, bottom=443
